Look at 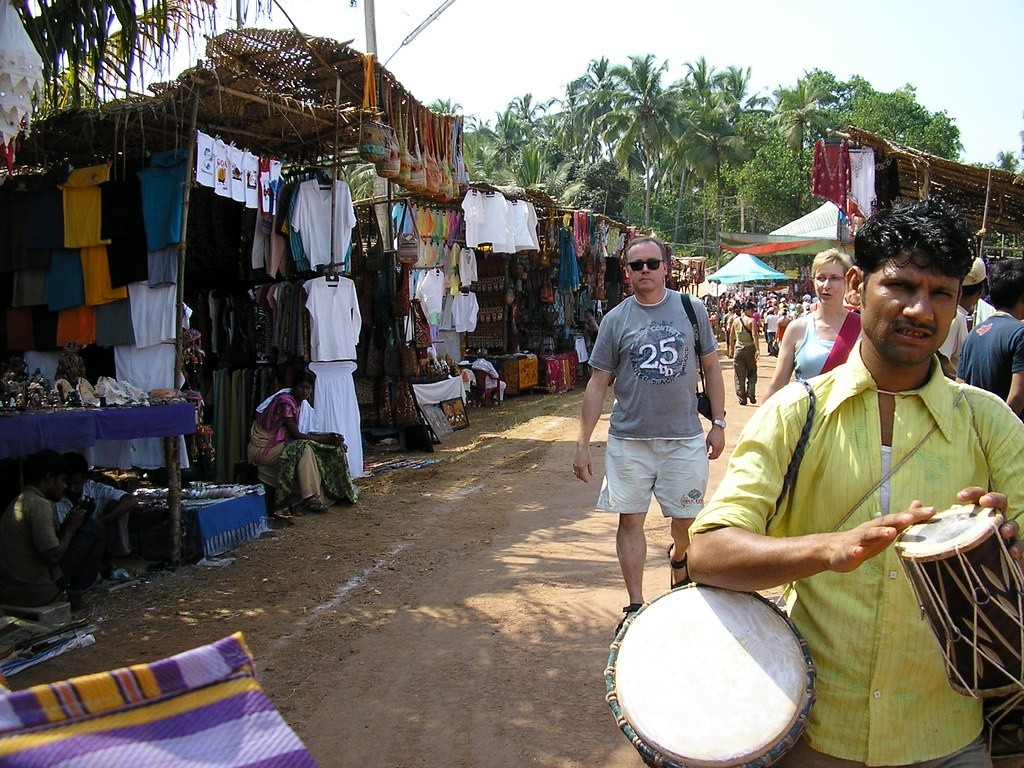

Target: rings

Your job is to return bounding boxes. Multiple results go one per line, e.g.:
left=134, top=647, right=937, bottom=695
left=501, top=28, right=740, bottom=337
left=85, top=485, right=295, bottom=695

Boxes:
left=573, top=470, right=575, bottom=473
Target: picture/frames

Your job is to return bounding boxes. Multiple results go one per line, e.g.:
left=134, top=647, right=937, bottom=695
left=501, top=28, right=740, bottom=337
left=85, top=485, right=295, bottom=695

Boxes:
left=439, top=397, right=470, bottom=431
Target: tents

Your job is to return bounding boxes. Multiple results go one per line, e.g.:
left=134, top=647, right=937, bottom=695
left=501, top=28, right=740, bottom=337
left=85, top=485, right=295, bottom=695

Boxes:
left=770, top=201, right=855, bottom=240
left=708, top=253, right=792, bottom=310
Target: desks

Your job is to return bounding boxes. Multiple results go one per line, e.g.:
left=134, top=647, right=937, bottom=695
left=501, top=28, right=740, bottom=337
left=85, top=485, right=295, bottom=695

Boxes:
left=0, top=406, right=194, bottom=568
left=466, top=352, right=577, bottom=401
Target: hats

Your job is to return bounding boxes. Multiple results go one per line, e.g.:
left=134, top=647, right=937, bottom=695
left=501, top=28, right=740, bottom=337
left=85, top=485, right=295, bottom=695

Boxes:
left=780, top=298, right=786, bottom=302
left=588, top=308, right=594, bottom=316
left=767, top=300, right=772, bottom=304
left=962, top=257, right=988, bottom=284
left=292, top=362, right=316, bottom=386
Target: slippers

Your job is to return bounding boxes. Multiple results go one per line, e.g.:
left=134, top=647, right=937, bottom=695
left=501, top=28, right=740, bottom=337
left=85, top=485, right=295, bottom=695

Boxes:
left=305, top=496, right=327, bottom=512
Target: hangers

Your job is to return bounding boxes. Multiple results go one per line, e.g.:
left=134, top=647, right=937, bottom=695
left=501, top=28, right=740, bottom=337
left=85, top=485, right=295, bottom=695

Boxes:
left=409, top=262, right=447, bottom=279
left=454, top=282, right=476, bottom=299
left=282, top=162, right=349, bottom=193
left=209, top=262, right=353, bottom=291
left=465, top=180, right=533, bottom=205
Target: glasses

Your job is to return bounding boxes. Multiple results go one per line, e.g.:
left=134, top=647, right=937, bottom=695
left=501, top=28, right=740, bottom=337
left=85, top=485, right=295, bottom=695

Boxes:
left=626, top=259, right=665, bottom=271
left=748, top=307, right=757, bottom=312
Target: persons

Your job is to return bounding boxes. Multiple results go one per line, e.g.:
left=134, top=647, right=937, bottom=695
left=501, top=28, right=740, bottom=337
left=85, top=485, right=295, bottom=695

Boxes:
left=472, top=348, right=506, bottom=404
left=584, top=309, right=598, bottom=353
left=53, top=452, right=135, bottom=580
left=0, top=449, right=106, bottom=615
left=762, top=248, right=863, bottom=405
left=689, top=198, right=1024, bottom=768
left=247, top=373, right=343, bottom=518
left=573, top=237, right=726, bottom=637
left=729, top=302, right=760, bottom=404
left=936, top=257, right=987, bottom=379
left=699, top=289, right=812, bottom=356
left=956, top=259, right=1024, bottom=423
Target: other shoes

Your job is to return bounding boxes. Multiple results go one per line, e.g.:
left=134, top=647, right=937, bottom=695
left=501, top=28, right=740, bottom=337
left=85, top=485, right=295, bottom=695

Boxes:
left=740, top=400, right=746, bottom=405
left=748, top=394, right=756, bottom=403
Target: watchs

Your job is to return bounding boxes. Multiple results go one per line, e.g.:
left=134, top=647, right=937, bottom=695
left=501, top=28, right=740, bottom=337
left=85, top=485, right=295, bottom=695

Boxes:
left=712, top=419, right=726, bottom=429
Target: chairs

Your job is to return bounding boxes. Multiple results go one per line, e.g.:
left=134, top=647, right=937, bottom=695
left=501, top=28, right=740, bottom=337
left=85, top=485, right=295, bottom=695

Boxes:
left=469, top=370, right=500, bottom=408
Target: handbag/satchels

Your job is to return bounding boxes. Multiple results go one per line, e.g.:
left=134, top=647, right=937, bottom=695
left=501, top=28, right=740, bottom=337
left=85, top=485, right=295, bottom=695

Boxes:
left=769, top=338, right=779, bottom=355
left=587, top=322, right=598, bottom=344
left=682, top=294, right=727, bottom=422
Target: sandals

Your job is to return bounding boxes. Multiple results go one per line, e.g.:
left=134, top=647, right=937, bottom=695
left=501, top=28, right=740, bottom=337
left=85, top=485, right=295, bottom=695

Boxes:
left=615, top=604, right=641, bottom=638
left=668, top=544, right=693, bottom=590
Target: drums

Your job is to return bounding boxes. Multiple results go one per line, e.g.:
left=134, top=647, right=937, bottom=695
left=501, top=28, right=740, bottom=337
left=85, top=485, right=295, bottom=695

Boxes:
left=604, top=581, right=818, bottom=768
left=895, top=502, right=1024, bottom=698
